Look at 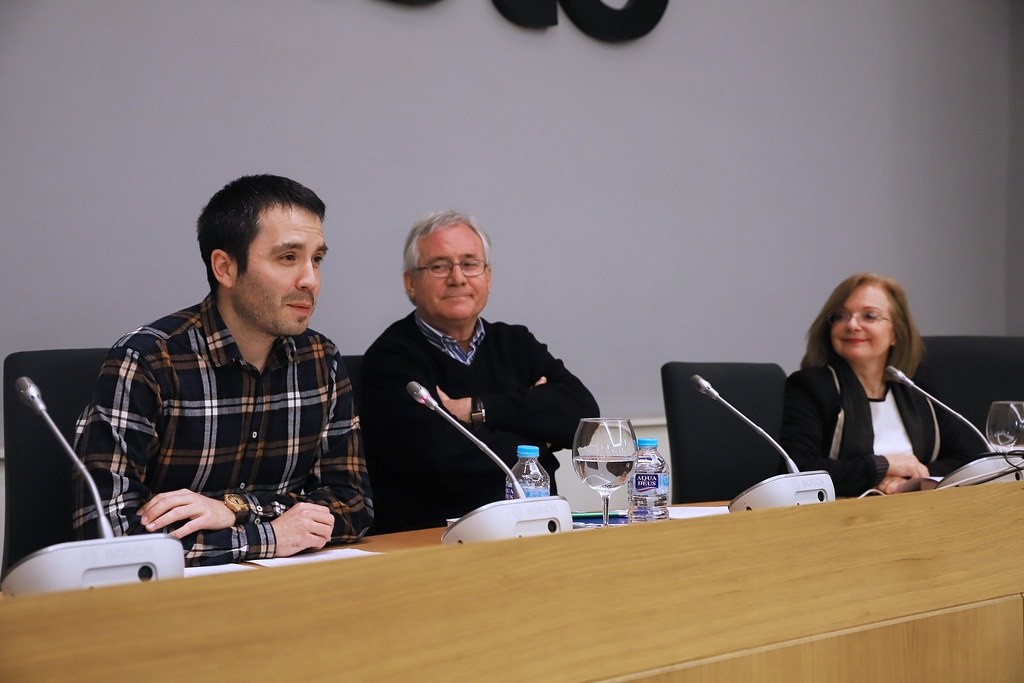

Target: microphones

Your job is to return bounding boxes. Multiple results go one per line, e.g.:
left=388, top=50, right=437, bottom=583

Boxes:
left=0, top=377, right=186, bottom=593
left=884, top=366, right=1024, bottom=489
left=689, top=374, right=835, bottom=513
left=405, top=382, right=573, bottom=544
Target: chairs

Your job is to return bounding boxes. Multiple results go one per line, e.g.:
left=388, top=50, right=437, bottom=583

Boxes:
left=1, top=335, right=1024, bottom=580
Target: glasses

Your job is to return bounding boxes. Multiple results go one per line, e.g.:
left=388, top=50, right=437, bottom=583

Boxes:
left=416, top=256, right=488, bottom=278
left=826, top=307, right=894, bottom=328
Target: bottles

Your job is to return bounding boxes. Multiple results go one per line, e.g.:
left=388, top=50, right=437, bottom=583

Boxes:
left=505, top=445, right=550, bottom=500
left=628, top=438, right=670, bottom=522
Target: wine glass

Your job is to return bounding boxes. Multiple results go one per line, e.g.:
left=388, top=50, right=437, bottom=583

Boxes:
left=572, top=418, right=637, bottom=527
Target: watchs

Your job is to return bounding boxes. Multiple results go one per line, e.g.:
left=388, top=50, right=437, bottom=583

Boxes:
left=224, top=493, right=250, bottom=526
left=471, top=396, right=484, bottom=424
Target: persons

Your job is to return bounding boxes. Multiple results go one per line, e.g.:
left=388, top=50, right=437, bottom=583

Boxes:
left=72, top=174, right=374, bottom=568
left=359, top=209, right=601, bottom=537
left=778, top=272, right=967, bottom=497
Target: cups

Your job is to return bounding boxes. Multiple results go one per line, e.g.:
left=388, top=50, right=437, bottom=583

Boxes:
left=985, top=401, right=1024, bottom=453
